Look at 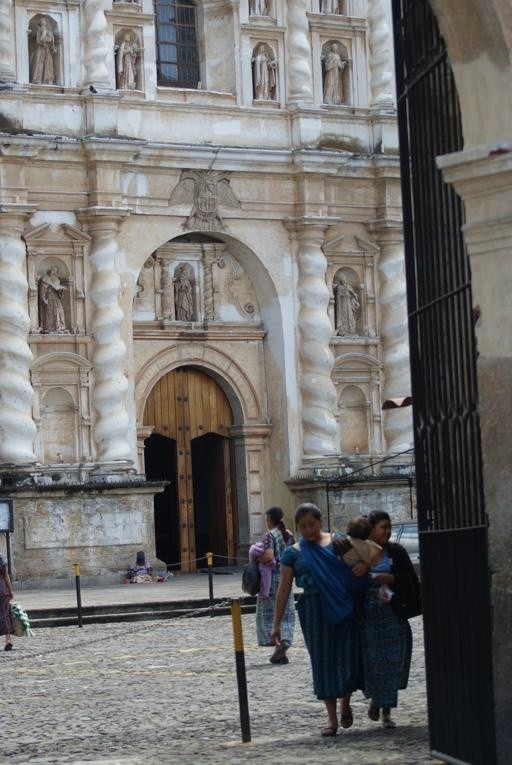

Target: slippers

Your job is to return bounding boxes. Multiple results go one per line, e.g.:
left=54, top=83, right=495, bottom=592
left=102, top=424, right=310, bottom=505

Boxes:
left=382, top=718, right=396, bottom=729
left=322, top=719, right=338, bottom=737
left=368, top=701, right=380, bottom=720
left=341, top=707, right=353, bottom=728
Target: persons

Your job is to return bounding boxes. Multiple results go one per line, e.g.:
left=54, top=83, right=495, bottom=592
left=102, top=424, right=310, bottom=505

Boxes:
left=253, top=44, right=276, bottom=101
left=250, top=0, right=268, bottom=16
left=116, top=33, right=138, bottom=91
left=335, top=273, right=359, bottom=337
left=323, top=43, right=347, bottom=105
left=0, top=553, right=13, bottom=651
left=174, top=271, right=193, bottom=321
left=254, top=507, right=295, bottom=664
left=321, top=0, right=339, bottom=14
left=270, top=502, right=371, bottom=736
left=333, top=518, right=384, bottom=566
left=38, top=266, right=67, bottom=334
left=359, top=510, right=422, bottom=729
left=128, top=551, right=153, bottom=576
left=27, top=17, right=57, bottom=87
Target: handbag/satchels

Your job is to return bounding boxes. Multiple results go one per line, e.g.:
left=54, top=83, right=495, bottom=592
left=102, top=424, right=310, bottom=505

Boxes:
left=10, top=601, right=29, bottom=638
left=241, top=559, right=261, bottom=596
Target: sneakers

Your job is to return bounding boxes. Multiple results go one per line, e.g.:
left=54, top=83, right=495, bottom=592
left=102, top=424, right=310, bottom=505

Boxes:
left=4, top=643, right=12, bottom=651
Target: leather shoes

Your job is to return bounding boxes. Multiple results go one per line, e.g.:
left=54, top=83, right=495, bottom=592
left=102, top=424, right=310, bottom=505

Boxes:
left=270, top=640, right=291, bottom=663
